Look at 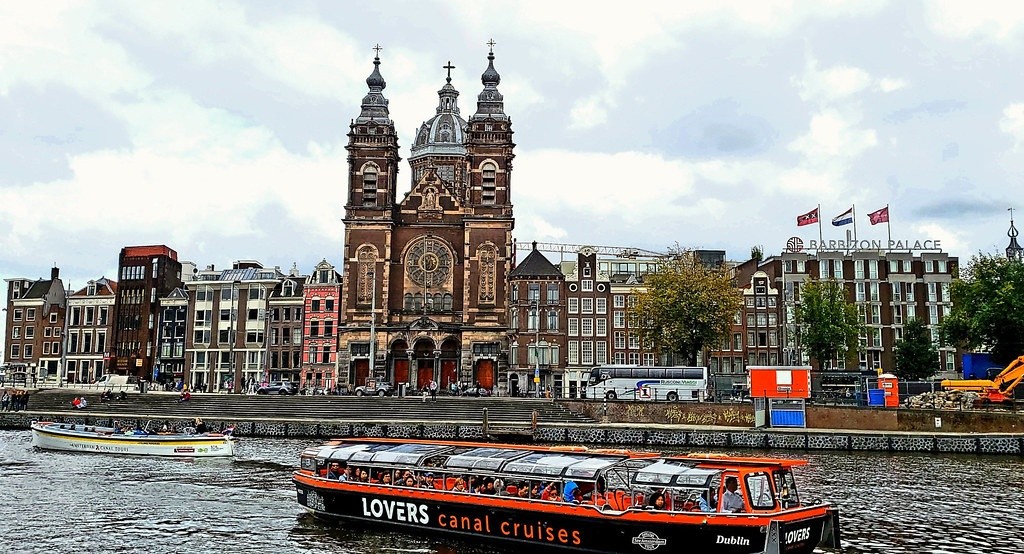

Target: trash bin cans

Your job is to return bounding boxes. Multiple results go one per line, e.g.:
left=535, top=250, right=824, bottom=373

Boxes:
left=868, top=389, right=885, bottom=406
left=398, top=382, right=406, bottom=399
left=140, top=380, right=147, bottom=393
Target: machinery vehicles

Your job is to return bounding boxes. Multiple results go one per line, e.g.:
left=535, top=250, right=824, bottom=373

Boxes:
left=942, top=355, right=1023, bottom=410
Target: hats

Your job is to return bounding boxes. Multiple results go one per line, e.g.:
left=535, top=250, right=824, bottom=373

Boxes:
left=426, top=471, right=436, bottom=478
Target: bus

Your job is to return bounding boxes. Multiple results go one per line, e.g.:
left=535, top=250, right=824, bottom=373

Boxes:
left=581, top=365, right=709, bottom=404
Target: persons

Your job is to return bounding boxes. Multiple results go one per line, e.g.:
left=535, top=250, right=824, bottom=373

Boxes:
left=73, top=396, right=87, bottom=409
left=326, top=458, right=445, bottom=489
left=699, top=486, right=718, bottom=512
left=185, top=420, right=209, bottom=434
left=163, top=420, right=169, bottom=432
left=451, top=474, right=605, bottom=505
left=651, top=479, right=671, bottom=510
left=421, top=379, right=437, bottom=403
left=720, top=477, right=744, bottom=513
left=717, top=486, right=726, bottom=498
left=240, top=374, right=255, bottom=393
left=649, top=493, right=665, bottom=510
left=1, top=389, right=29, bottom=412
left=457, top=380, right=463, bottom=395
left=115, top=423, right=159, bottom=435
left=166, top=380, right=190, bottom=402
left=100, top=389, right=127, bottom=403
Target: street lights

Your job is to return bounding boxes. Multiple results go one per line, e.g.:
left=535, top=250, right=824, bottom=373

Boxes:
left=227, top=280, right=243, bottom=396
left=466, top=366, right=470, bottom=387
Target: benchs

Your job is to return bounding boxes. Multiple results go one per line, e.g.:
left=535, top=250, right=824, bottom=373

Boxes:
left=320, top=468, right=467, bottom=491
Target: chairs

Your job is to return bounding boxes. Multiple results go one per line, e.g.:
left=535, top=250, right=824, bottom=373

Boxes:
left=603, top=489, right=619, bottom=510
left=622, top=494, right=632, bottom=511
left=506, top=483, right=518, bottom=496
left=614, top=487, right=626, bottom=511
left=592, top=492, right=606, bottom=506
left=663, top=492, right=671, bottom=511
left=634, top=492, right=644, bottom=506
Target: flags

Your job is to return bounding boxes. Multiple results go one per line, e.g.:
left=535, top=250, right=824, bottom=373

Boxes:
left=222, top=426, right=234, bottom=435
left=797, top=208, right=818, bottom=226
left=867, top=206, right=889, bottom=225
left=832, top=207, right=853, bottom=226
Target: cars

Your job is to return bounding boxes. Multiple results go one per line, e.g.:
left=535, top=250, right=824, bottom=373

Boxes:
left=257, top=381, right=298, bottom=395
left=355, top=381, right=395, bottom=397
left=461, top=387, right=477, bottom=396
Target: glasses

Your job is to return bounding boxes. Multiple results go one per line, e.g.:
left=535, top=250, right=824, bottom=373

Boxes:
left=534, top=488, right=539, bottom=491
left=331, top=464, right=340, bottom=467
left=550, top=492, right=558, bottom=496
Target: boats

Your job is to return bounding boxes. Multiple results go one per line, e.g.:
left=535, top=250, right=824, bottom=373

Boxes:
left=31, top=420, right=236, bottom=457
left=293, top=438, right=831, bottom=553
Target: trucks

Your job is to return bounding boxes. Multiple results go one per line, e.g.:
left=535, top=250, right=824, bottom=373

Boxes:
left=94, top=374, right=138, bottom=386
